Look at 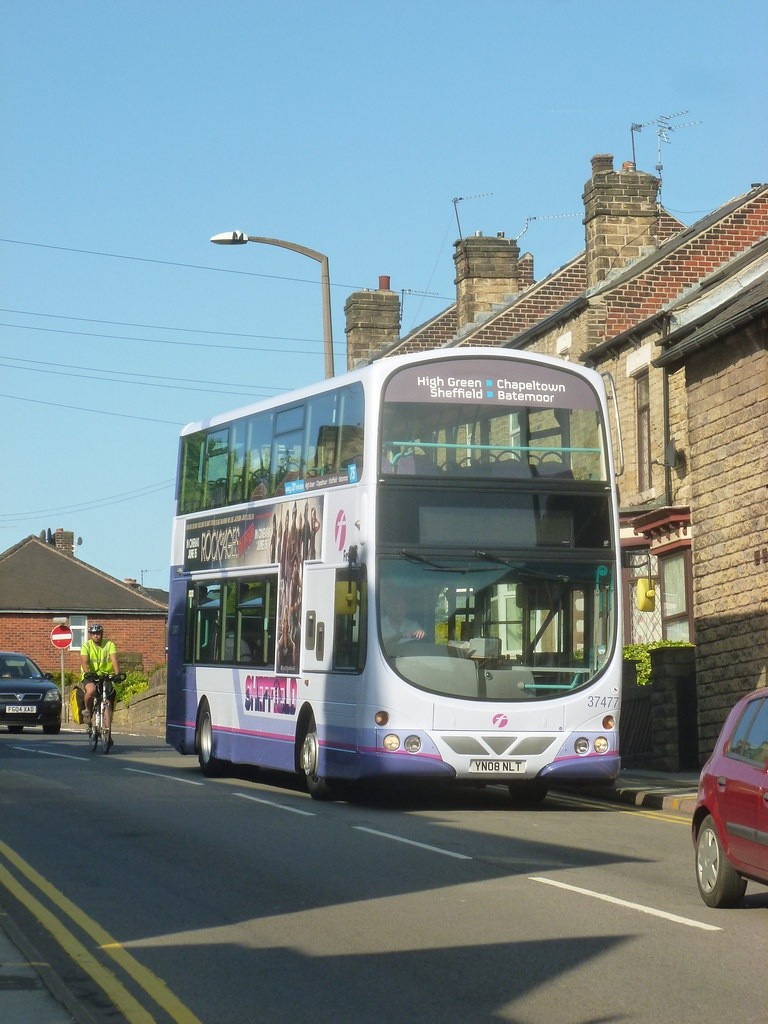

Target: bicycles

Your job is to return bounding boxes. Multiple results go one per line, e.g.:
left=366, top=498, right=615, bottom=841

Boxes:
left=82, top=672, right=127, bottom=754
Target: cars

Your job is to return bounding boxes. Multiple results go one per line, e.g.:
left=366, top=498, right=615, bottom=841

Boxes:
left=684, top=687, right=768, bottom=910
left=0, top=651, right=62, bottom=735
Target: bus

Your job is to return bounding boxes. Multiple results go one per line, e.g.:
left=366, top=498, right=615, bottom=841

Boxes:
left=165, top=347, right=658, bottom=806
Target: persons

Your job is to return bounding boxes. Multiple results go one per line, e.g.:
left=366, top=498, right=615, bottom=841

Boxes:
left=78, top=624, right=120, bottom=746
left=269, top=501, right=321, bottom=672
left=380, top=591, right=426, bottom=660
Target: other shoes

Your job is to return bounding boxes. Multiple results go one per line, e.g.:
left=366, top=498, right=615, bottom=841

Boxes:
left=81, top=709, right=92, bottom=724
left=109, top=738, right=114, bottom=746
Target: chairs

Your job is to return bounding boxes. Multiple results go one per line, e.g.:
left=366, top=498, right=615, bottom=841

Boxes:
left=189, top=447, right=576, bottom=511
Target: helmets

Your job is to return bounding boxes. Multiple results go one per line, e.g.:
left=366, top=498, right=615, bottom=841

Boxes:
left=88, top=623, right=103, bottom=632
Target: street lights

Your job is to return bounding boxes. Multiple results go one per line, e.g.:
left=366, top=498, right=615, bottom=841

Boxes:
left=209, top=229, right=337, bottom=383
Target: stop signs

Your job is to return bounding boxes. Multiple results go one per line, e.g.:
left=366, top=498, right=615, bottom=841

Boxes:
left=50, top=624, right=74, bottom=650
